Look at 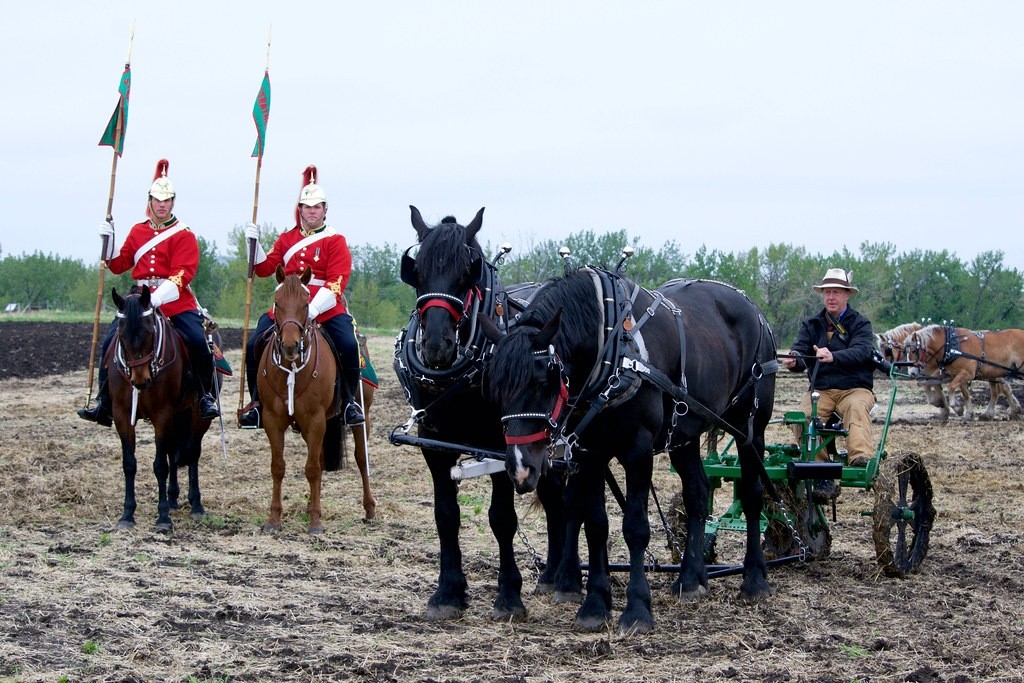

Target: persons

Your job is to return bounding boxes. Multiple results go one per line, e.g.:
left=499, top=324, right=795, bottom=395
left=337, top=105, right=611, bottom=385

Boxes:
left=239, top=167, right=366, bottom=427
left=76, top=159, right=220, bottom=426
left=780, top=267, right=877, bottom=498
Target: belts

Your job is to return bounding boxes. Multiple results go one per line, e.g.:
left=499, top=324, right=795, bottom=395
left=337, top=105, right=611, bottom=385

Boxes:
left=306, top=279, right=326, bottom=286
left=137, top=279, right=166, bottom=287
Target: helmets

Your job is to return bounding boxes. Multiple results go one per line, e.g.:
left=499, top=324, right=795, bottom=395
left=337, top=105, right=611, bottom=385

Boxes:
left=148, top=159, right=175, bottom=218
left=298, top=166, right=328, bottom=224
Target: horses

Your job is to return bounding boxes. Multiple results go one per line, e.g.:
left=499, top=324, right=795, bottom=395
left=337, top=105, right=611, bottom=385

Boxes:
left=405, top=202, right=544, bottom=615
left=257, top=261, right=385, bottom=536
left=875, top=318, right=1024, bottom=422
left=100, top=282, right=216, bottom=536
left=499, top=264, right=786, bottom=638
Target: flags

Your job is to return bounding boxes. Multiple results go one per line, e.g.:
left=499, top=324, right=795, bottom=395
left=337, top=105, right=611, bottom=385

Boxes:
left=98, top=68, right=131, bottom=158
left=250, top=73, right=271, bottom=158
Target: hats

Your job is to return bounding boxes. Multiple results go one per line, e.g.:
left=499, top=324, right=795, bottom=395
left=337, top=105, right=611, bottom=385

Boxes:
left=812, top=268, right=859, bottom=295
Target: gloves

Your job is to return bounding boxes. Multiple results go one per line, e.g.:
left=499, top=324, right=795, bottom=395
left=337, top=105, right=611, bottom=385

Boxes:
left=245, top=222, right=267, bottom=264
left=98, top=219, right=115, bottom=260
left=150, top=280, right=179, bottom=308
left=308, top=287, right=337, bottom=320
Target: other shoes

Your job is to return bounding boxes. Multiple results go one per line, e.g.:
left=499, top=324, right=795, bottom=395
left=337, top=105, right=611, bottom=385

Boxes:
left=813, top=479, right=835, bottom=499
left=850, top=456, right=869, bottom=467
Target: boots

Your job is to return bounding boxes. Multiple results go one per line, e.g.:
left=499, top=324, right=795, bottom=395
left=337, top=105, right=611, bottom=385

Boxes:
left=239, top=369, right=264, bottom=428
left=338, top=367, right=366, bottom=424
left=192, top=352, right=219, bottom=419
left=76, top=378, right=112, bottom=428
